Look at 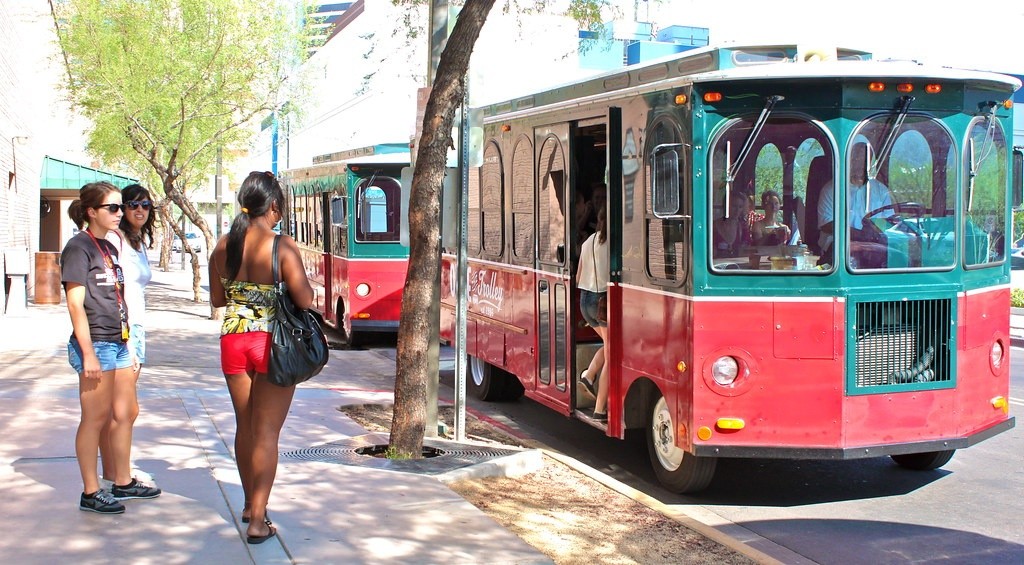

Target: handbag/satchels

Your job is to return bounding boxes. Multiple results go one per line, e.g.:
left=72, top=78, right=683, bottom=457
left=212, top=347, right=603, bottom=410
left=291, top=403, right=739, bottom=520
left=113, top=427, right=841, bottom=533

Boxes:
left=265, top=235, right=329, bottom=388
left=596, top=296, right=606, bottom=324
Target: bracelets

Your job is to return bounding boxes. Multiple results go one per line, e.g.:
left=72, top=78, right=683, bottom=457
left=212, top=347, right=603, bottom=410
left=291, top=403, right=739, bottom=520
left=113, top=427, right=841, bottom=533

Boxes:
left=580, top=232, right=588, bottom=236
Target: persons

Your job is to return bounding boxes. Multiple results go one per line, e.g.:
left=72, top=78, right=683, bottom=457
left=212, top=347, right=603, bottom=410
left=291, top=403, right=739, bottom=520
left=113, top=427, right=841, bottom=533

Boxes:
left=747, top=188, right=793, bottom=247
left=209, top=171, right=315, bottom=543
left=94, top=182, right=156, bottom=490
left=707, top=194, right=746, bottom=256
left=818, top=142, right=897, bottom=264
left=742, top=189, right=765, bottom=239
left=575, top=207, right=608, bottom=424
left=571, top=182, right=608, bottom=279
left=58, top=181, right=164, bottom=513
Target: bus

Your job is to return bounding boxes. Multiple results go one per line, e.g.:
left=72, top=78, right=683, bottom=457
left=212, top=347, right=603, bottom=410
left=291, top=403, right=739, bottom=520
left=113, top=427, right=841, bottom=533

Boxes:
left=276, top=143, right=416, bottom=348
left=467, top=41, right=1017, bottom=496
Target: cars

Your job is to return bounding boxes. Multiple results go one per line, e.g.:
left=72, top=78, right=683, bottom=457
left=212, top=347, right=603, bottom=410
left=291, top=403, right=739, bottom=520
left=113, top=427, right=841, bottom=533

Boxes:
left=171, top=231, right=203, bottom=253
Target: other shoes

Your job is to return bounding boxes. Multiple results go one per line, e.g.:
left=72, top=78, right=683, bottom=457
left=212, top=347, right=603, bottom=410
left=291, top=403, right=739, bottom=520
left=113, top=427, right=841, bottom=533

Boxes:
left=578, top=378, right=596, bottom=400
left=592, top=411, right=607, bottom=422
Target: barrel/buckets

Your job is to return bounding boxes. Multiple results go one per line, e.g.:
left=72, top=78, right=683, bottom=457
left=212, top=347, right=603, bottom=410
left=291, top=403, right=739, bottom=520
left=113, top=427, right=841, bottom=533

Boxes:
left=33, top=252, right=63, bottom=304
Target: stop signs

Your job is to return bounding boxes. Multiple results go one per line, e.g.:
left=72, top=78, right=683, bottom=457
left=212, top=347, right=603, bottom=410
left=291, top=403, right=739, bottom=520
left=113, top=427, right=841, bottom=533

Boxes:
left=223, top=221, right=228, bottom=227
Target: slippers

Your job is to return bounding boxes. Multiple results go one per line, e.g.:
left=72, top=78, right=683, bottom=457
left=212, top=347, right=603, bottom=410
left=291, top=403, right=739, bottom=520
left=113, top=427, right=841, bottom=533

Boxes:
left=242, top=516, right=249, bottom=523
left=246, top=525, right=275, bottom=544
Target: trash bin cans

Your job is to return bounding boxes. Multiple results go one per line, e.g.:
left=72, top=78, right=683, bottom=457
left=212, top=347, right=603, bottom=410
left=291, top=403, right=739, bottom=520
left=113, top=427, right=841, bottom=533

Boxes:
left=35, top=252, right=62, bottom=304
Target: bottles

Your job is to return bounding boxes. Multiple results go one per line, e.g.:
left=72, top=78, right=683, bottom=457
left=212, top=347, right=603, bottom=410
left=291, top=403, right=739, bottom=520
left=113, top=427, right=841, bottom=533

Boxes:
left=802, top=251, right=813, bottom=270
left=797, top=244, right=808, bottom=270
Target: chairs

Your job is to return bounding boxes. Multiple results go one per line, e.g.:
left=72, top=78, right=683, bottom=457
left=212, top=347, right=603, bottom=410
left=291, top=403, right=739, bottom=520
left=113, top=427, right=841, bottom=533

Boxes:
left=805, top=155, right=832, bottom=256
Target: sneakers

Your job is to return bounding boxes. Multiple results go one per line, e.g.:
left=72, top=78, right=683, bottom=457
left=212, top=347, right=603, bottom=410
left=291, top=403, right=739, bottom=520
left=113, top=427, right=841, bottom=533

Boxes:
left=79, top=489, right=125, bottom=513
left=112, top=478, right=161, bottom=499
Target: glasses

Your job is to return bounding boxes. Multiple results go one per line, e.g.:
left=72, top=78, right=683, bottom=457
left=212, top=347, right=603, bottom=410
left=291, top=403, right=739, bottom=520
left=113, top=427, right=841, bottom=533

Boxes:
left=250, top=171, right=275, bottom=180
left=94, top=204, right=126, bottom=212
left=126, top=200, right=152, bottom=210
